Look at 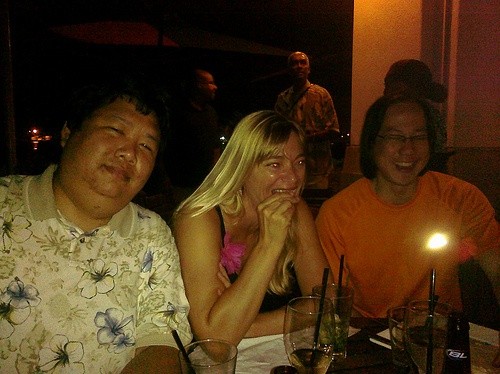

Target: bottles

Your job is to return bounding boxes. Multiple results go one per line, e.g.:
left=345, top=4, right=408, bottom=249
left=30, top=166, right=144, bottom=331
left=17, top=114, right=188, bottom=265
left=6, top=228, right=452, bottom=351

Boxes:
left=442, top=312, right=471, bottom=374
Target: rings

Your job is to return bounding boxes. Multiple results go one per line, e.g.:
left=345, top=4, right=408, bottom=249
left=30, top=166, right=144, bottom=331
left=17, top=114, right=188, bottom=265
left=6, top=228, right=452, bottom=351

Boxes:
left=268, top=201, right=280, bottom=210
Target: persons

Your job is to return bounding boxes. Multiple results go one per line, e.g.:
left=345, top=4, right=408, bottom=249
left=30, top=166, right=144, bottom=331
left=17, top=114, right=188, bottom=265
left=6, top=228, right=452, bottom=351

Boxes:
left=315, top=93, right=500, bottom=319
left=167, top=109, right=335, bottom=362
left=274, top=51, right=341, bottom=218
left=383, top=59, right=447, bottom=173
left=169, top=67, right=222, bottom=193
left=0, top=78, right=191, bottom=374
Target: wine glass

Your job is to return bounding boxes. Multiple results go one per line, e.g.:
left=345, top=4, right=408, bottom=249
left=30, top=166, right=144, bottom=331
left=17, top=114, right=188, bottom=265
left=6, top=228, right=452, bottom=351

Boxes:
left=404, top=300, right=451, bottom=373
left=283, top=295, right=336, bottom=374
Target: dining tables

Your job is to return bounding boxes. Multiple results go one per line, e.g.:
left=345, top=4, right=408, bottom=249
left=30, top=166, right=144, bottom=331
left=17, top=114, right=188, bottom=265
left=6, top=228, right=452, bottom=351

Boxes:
left=188, top=317, right=499, bottom=374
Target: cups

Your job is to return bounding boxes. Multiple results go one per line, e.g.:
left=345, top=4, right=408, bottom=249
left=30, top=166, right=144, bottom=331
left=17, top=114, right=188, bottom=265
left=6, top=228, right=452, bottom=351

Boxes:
left=387, top=306, right=424, bottom=367
left=178, top=339, right=238, bottom=374
left=312, top=283, right=354, bottom=362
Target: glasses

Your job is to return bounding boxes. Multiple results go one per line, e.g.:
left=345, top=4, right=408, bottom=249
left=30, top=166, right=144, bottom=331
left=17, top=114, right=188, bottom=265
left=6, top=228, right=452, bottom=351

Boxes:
left=377, top=134, right=430, bottom=146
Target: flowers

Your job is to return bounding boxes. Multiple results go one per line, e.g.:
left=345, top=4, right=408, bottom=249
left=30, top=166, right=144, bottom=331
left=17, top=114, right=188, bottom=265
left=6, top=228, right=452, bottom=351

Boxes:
left=220, top=229, right=246, bottom=277
